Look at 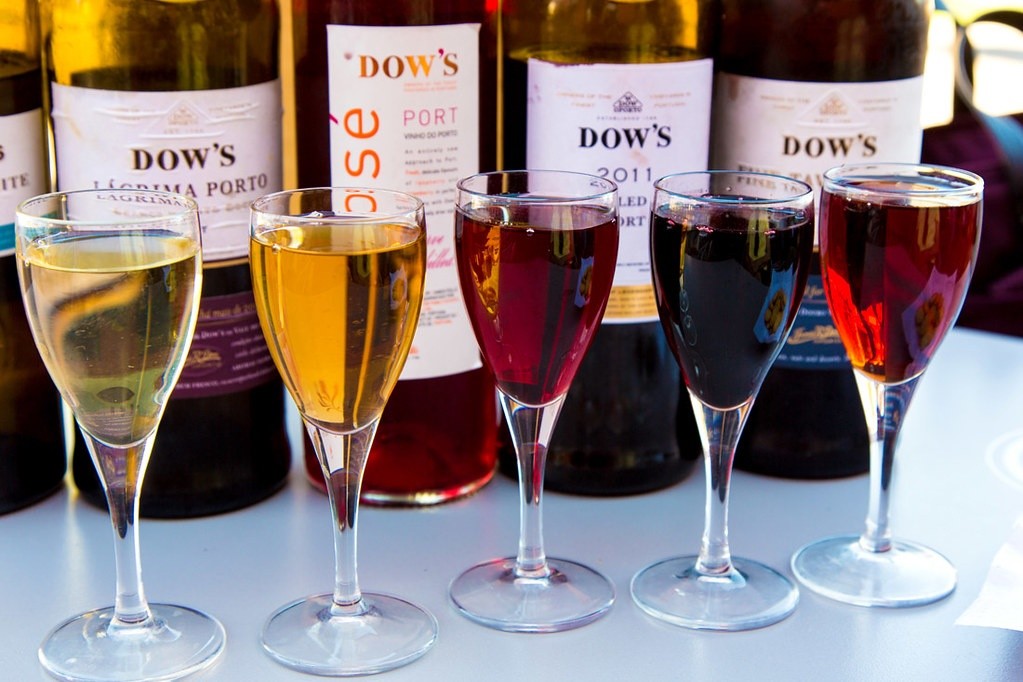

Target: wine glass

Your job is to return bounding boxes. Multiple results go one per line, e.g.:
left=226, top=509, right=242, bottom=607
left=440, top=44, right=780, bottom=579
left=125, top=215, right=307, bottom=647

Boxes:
left=250, top=186, right=439, bottom=677
left=449, top=170, right=619, bottom=632
left=16, top=189, right=226, bottom=682
left=629, top=171, right=815, bottom=631
left=790, top=163, right=985, bottom=606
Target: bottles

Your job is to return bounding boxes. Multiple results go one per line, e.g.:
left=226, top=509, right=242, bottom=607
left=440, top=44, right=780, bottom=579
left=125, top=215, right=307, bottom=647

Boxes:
left=697, top=0, right=930, bottom=483
left=0, top=0, right=66, bottom=516
left=45, top=0, right=291, bottom=521
left=498, top=0, right=716, bottom=498
left=291, top=0, right=497, bottom=507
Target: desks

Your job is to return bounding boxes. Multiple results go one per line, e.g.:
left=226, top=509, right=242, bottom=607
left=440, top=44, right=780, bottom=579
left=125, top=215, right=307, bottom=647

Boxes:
left=0, top=327, right=1023, bottom=682
